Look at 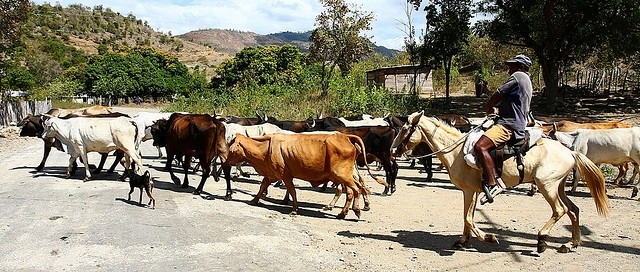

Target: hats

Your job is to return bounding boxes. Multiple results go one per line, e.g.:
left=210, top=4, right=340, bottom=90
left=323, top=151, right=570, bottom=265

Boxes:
left=504, top=54, right=532, bottom=69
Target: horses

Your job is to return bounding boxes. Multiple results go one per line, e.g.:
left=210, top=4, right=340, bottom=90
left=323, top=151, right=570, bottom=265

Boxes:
left=390, top=110, right=611, bottom=254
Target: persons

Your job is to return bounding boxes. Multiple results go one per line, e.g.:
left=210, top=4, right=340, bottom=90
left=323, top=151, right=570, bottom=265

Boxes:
left=474, top=54, right=533, bottom=205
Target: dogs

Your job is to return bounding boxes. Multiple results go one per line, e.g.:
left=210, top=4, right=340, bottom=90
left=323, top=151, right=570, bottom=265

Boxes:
left=121, top=168, right=156, bottom=209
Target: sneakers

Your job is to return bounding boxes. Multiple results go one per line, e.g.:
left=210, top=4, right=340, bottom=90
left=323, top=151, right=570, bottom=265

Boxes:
left=480, top=184, right=503, bottom=205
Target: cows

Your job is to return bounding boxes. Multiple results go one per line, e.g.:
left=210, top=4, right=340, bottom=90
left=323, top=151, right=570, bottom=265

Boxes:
left=253, top=109, right=307, bottom=188
left=145, top=112, right=233, bottom=201
left=211, top=108, right=283, bottom=178
left=41, top=116, right=144, bottom=182
left=538, top=121, right=632, bottom=186
left=18, top=112, right=138, bottom=176
left=226, top=115, right=260, bottom=125
left=132, top=111, right=172, bottom=159
left=340, top=113, right=390, bottom=170
left=548, top=122, right=640, bottom=199
left=384, top=113, right=471, bottom=180
left=45, top=106, right=113, bottom=118
left=225, top=132, right=389, bottom=220
left=302, top=116, right=398, bottom=193
